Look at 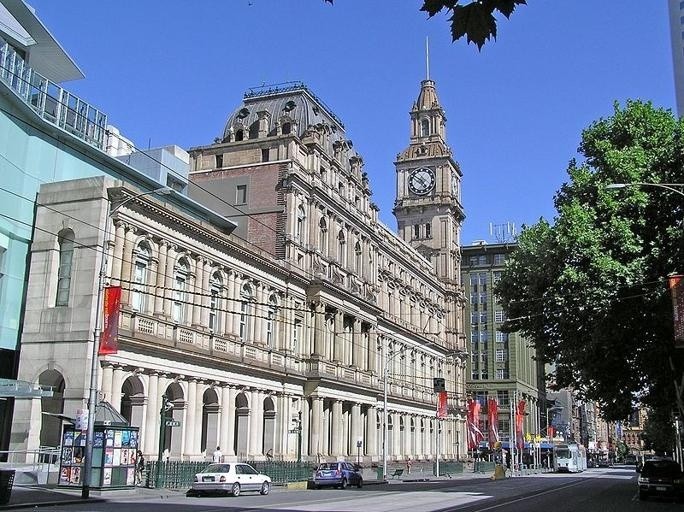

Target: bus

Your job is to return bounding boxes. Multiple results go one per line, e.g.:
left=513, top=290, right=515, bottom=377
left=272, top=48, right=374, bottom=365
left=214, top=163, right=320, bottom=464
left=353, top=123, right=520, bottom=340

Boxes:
left=554, top=444, right=588, bottom=473
left=587, top=449, right=609, bottom=468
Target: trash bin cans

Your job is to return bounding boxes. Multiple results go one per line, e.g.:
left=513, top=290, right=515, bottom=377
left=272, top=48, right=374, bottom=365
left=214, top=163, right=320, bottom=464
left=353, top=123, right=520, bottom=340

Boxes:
left=377, top=466, right=383, bottom=480
left=0, top=470, right=15, bottom=505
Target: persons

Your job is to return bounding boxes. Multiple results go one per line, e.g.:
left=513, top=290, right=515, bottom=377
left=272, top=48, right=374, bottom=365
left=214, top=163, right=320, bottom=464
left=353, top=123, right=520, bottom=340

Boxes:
left=212, top=446, right=222, bottom=463
left=136, top=450, right=144, bottom=486
left=407, top=458, right=412, bottom=474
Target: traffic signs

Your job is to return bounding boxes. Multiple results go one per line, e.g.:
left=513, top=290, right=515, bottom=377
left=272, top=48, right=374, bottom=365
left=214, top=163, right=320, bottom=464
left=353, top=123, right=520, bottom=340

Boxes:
left=433, top=377, right=445, bottom=392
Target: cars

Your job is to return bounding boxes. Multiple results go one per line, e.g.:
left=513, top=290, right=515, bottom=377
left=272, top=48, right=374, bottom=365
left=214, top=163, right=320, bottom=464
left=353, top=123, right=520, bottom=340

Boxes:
left=636, top=461, right=683, bottom=503
left=193, top=463, right=273, bottom=497
left=314, top=461, right=363, bottom=489
left=624, top=458, right=634, bottom=464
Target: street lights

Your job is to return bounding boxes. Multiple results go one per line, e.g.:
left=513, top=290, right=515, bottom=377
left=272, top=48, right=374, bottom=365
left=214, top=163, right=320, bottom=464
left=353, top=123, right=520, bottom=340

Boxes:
left=82, top=187, right=175, bottom=499
left=436, top=352, right=470, bottom=478
left=383, top=341, right=435, bottom=481
left=546, top=406, right=564, bottom=469
